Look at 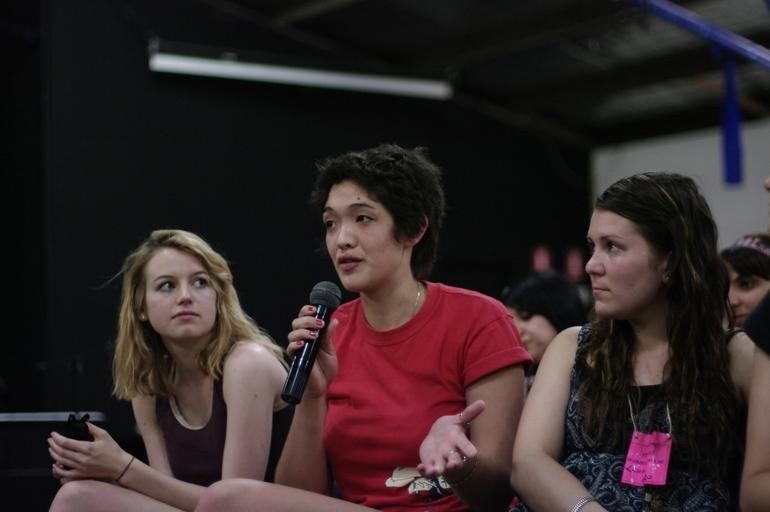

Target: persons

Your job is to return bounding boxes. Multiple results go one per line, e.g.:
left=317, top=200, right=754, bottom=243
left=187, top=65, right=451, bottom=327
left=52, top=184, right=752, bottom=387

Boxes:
left=46, top=227, right=292, bottom=512
left=194, top=140, right=532, bottom=512
left=498, top=270, right=587, bottom=398
left=739, top=270, right=769, bottom=509
left=716, top=232, right=770, bottom=329
left=511, top=169, right=769, bottom=511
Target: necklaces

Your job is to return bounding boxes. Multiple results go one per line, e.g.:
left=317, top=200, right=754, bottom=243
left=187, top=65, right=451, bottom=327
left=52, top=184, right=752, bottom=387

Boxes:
left=410, top=279, right=421, bottom=317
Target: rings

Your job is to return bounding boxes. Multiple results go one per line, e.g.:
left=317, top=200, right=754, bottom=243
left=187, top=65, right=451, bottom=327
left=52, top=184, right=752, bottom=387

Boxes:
left=444, top=448, right=458, bottom=458
left=457, top=410, right=469, bottom=424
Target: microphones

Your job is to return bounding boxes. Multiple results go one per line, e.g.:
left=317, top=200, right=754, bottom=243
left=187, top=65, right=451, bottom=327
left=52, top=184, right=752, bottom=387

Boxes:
left=279, top=280, right=343, bottom=405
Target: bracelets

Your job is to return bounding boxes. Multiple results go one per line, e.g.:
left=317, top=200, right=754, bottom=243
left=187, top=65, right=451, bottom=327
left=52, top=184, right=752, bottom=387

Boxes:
left=569, top=495, right=599, bottom=512
left=110, top=454, right=134, bottom=485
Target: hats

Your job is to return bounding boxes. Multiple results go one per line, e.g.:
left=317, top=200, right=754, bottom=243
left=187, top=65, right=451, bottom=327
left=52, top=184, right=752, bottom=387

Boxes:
left=720, top=234, right=769, bottom=277
